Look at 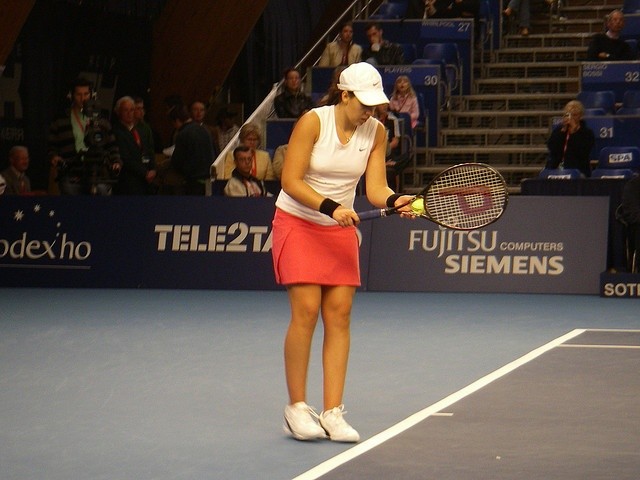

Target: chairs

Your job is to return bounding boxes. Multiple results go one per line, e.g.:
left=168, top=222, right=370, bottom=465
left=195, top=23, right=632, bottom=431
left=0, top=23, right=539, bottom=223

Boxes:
left=523, top=0, right=640, bottom=207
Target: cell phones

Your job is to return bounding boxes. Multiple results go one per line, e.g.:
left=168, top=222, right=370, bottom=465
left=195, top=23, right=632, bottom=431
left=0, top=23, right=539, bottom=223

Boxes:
left=566, top=112, right=570, bottom=118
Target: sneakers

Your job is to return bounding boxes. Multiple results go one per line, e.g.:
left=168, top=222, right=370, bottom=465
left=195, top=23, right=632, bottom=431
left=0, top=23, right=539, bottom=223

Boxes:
left=282, top=401, right=326, bottom=441
left=319, top=404, right=360, bottom=442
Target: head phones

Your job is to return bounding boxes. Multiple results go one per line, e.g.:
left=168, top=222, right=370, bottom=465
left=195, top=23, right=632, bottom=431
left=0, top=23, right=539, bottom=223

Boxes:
left=66, top=79, right=98, bottom=100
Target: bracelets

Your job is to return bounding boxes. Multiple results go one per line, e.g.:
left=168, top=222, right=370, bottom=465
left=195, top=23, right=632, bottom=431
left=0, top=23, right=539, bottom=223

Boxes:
left=386, top=193, right=406, bottom=214
left=320, top=198, right=342, bottom=219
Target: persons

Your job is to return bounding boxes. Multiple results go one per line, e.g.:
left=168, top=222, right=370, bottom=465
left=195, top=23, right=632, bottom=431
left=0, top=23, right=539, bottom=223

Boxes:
left=272, top=62, right=420, bottom=443
left=224, top=122, right=271, bottom=180
left=213, top=107, right=240, bottom=154
left=317, top=65, right=346, bottom=106
left=274, top=68, right=316, bottom=118
left=317, top=20, right=365, bottom=67
left=363, top=21, right=405, bottom=64
left=422, top=0, right=442, bottom=19
left=1, top=146, right=31, bottom=196
left=540, top=100, right=597, bottom=177
left=46, top=78, right=124, bottom=177
left=372, top=103, right=401, bottom=162
left=175, top=101, right=220, bottom=157
left=445, top=0, right=482, bottom=47
left=389, top=75, right=420, bottom=129
left=135, top=101, right=154, bottom=163
left=504, top=0, right=531, bottom=36
left=586, top=9, right=633, bottom=60
left=111, top=96, right=156, bottom=183
left=272, top=144, right=288, bottom=181
left=224, top=145, right=266, bottom=198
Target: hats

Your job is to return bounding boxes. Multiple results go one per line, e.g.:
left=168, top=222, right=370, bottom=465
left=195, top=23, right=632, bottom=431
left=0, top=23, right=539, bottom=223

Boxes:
left=336, top=61, right=390, bottom=107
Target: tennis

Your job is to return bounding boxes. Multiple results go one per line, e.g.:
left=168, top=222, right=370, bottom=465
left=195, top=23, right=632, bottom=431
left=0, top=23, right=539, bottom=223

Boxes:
left=411, top=198, right=426, bottom=215
